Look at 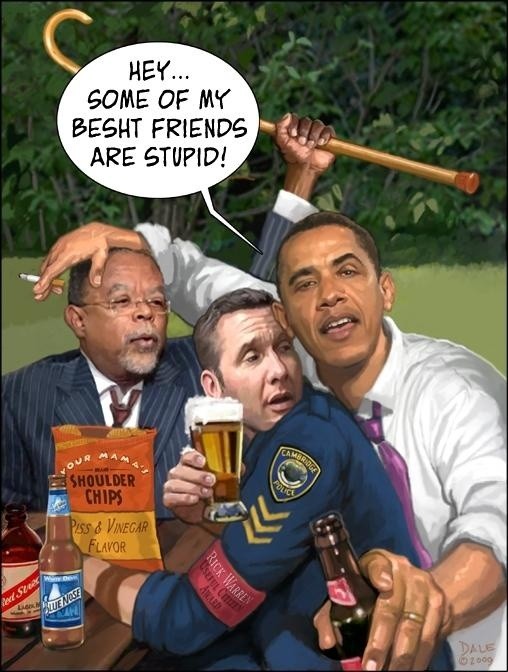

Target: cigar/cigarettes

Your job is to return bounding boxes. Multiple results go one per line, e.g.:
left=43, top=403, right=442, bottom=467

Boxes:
left=18, top=272, right=64, bottom=287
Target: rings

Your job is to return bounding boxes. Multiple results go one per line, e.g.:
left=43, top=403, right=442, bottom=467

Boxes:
left=400, top=609, right=425, bottom=624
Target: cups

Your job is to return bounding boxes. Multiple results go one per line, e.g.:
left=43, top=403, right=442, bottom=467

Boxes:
left=187, top=400, right=249, bottom=526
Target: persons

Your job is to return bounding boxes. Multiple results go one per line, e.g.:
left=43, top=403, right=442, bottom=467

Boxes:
left=0, top=111, right=334, bottom=519
left=30, top=210, right=507, bottom=671
left=80, top=286, right=460, bottom=671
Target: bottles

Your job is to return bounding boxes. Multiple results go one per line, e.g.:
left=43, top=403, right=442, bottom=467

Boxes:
left=1, top=475, right=85, bottom=652
left=307, top=509, right=395, bottom=672
